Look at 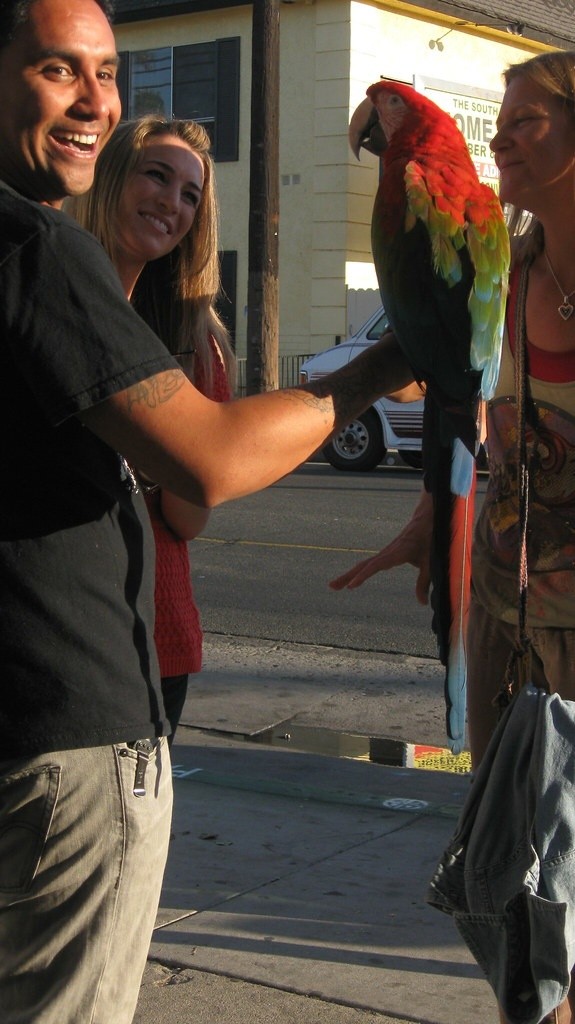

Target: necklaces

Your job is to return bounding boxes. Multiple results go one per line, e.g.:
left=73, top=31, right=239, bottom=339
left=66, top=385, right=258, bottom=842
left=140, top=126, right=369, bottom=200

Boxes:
left=544, top=248, right=575, bottom=321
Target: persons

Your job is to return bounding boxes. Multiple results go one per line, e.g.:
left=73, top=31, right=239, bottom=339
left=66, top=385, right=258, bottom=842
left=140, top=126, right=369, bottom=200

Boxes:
left=0, top=0, right=426, bottom=1024
left=328, top=51, right=575, bottom=1024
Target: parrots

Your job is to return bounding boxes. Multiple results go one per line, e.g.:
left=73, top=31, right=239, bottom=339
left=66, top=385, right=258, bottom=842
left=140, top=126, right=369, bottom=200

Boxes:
left=347, top=80, right=511, bottom=757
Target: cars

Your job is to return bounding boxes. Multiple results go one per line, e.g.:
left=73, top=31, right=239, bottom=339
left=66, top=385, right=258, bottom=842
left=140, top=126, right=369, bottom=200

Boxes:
left=299, top=304, right=425, bottom=473
